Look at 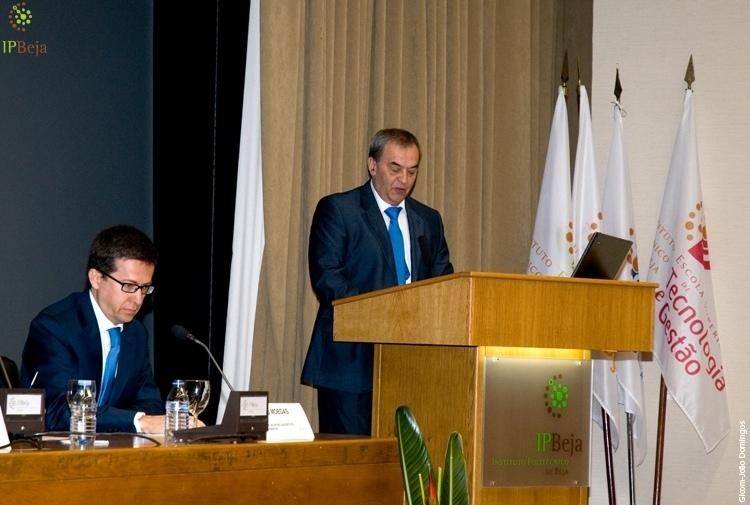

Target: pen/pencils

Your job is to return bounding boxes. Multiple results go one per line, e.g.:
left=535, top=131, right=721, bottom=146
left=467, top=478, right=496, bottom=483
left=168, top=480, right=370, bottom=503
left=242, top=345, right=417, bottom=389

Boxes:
left=28, top=371, right=39, bottom=390
left=0, top=356, right=13, bottom=391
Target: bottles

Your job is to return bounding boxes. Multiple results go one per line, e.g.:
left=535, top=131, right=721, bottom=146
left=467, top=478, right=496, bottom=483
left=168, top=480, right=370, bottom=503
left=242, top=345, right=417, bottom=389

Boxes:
left=68, top=379, right=98, bottom=444
left=165, top=379, right=190, bottom=441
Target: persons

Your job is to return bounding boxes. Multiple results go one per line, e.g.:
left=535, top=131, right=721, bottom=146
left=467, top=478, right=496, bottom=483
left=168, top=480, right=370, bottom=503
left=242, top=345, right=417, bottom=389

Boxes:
left=300, top=127, right=455, bottom=435
left=20, top=223, right=207, bottom=435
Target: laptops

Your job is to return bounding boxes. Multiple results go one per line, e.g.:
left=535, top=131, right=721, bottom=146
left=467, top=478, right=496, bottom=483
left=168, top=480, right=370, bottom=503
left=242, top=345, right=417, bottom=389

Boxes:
left=571, top=231, right=633, bottom=280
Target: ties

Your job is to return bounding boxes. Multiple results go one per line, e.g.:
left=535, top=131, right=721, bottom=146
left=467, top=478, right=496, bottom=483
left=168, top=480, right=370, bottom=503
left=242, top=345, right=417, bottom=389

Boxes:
left=98, top=327, right=120, bottom=407
left=385, top=207, right=410, bottom=285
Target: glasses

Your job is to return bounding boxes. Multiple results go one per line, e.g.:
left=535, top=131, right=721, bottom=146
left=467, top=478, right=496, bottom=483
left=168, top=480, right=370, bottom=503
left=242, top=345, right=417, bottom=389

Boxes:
left=105, top=274, right=155, bottom=294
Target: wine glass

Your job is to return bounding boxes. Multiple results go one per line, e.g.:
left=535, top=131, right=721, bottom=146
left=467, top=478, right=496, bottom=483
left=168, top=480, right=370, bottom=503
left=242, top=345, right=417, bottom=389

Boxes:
left=182, top=380, right=210, bottom=429
left=67, top=379, right=97, bottom=409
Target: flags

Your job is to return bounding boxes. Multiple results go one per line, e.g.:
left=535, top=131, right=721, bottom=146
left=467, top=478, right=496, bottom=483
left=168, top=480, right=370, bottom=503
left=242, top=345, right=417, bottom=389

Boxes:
left=601, top=100, right=647, bottom=468
left=524, top=83, right=574, bottom=277
left=572, top=81, right=620, bottom=451
left=643, top=88, right=732, bottom=455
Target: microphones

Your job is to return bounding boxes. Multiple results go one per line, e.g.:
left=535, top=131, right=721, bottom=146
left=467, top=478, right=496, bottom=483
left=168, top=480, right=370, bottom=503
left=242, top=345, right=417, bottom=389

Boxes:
left=172, top=325, right=269, bottom=444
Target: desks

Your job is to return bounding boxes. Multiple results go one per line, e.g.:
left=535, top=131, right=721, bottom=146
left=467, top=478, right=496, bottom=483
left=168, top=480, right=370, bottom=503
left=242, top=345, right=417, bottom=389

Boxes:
left=0, top=433, right=405, bottom=505
